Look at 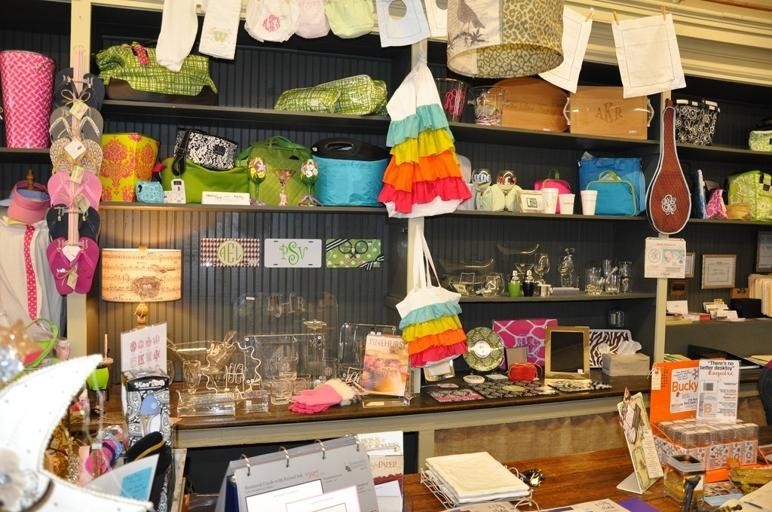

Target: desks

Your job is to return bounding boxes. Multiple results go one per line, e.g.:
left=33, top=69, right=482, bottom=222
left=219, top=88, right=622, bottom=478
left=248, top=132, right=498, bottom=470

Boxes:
left=378, top=427, right=769, bottom=512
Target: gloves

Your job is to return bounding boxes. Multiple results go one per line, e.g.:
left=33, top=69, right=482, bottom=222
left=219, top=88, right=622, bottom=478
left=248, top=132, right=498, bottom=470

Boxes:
left=288, top=377, right=357, bottom=414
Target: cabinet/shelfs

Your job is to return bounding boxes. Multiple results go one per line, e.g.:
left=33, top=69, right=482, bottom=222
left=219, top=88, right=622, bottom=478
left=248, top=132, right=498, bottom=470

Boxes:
left=0, top=0, right=772, bottom=468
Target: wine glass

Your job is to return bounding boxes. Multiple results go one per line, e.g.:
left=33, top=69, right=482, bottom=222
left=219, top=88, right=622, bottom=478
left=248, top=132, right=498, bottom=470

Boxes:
left=298, top=158, right=324, bottom=207
left=181, top=359, right=202, bottom=413
left=470, top=166, right=493, bottom=212
left=496, top=168, right=518, bottom=213
left=247, top=155, right=267, bottom=206
left=273, top=166, right=298, bottom=206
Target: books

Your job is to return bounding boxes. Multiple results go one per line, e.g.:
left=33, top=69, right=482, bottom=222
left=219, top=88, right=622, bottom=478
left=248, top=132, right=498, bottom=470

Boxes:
left=356, top=430, right=403, bottom=498
left=425, top=451, right=530, bottom=505
left=362, top=332, right=408, bottom=397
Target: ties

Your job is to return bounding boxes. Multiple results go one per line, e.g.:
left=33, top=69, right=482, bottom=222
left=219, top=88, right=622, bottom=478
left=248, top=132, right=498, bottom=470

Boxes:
left=23, top=224, right=37, bottom=321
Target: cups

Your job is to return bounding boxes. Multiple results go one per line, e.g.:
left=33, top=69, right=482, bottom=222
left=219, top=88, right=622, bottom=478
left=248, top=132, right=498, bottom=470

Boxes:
left=167, top=360, right=175, bottom=387
left=538, top=285, right=551, bottom=296
left=507, top=280, right=522, bottom=297
left=521, top=281, right=536, bottom=297
left=270, top=355, right=337, bottom=405
left=433, top=77, right=467, bottom=124
left=488, top=271, right=507, bottom=296
left=469, top=84, right=505, bottom=128
left=557, top=194, right=575, bottom=215
left=607, top=309, right=625, bottom=329
left=579, top=189, right=598, bottom=216
left=542, top=187, right=558, bottom=214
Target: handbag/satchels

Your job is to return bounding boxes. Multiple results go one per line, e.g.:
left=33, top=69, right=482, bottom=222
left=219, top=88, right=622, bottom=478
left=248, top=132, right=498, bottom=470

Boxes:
left=159, top=126, right=391, bottom=207
left=94, top=45, right=220, bottom=106
left=749, top=131, right=772, bottom=152
left=274, top=74, right=388, bottom=117
left=396, top=224, right=468, bottom=370
left=728, top=170, right=772, bottom=223
left=673, top=99, right=720, bottom=146
left=578, top=152, right=645, bottom=217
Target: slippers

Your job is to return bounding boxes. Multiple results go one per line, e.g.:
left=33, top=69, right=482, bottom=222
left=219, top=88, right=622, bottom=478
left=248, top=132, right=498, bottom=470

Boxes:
left=46, top=68, right=106, bottom=296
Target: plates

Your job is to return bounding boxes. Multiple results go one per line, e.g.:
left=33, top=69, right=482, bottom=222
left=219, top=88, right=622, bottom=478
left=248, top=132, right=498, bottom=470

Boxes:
left=462, top=325, right=506, bottom=373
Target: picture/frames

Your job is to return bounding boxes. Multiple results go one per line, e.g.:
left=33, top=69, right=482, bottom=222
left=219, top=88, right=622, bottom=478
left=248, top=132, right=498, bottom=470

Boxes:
left=542, top=325, right=590, bottom=380
